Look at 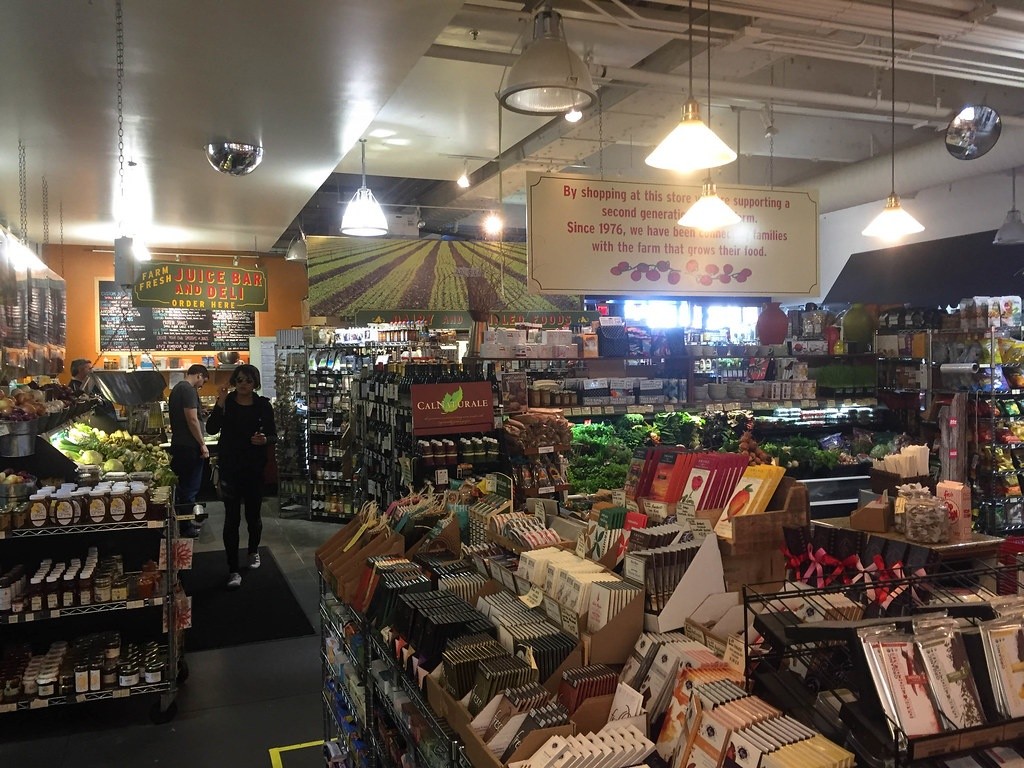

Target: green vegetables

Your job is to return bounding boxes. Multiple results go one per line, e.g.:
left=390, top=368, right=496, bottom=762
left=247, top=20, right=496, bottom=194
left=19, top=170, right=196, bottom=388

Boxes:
left=760, top=435, right=839, bottom=470
left=60, top=422, right=156, bottom=472
left=569, top=412, right=706, bottom=494
left=870, top=444, right=889, bottom=459
left=154, top=467, right=176, bottom=488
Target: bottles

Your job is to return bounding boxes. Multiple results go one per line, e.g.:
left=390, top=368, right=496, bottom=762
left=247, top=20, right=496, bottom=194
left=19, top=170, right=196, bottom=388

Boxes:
left=273, top=320, right=499, bottom=514
left=29, top=546, right=98, bottom=610
left=0, top=483, right=150, bottom=529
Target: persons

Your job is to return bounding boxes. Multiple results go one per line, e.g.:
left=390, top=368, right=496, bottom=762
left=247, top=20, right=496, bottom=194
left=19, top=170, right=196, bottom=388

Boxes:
left=168, top=365, right=209, bottom=539
left=68, top=359, right=92, bottom=390
left=206, top=364, right=277, bottom=587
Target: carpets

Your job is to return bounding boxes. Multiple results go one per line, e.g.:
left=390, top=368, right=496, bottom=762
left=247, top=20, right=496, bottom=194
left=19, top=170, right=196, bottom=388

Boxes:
left=179, top=545, right=314, bottom=654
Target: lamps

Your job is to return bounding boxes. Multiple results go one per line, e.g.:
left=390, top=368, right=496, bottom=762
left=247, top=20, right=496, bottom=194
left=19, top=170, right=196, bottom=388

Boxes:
left=340, top=137, right=390, bottom=239
left=646, top=0, right=742, bottom=183
left=991, top=167, right=1024, bottom=248
left=675, top=0, right=744, bottom=235
left=285, top=208, right=308, bottom=263
left=495, top=0, right=598, bottom=117
left=457, top=158, right=470, bottom=188
left=860, top=0, right=929, bottom=243
left=757, top=99, right=783, bottom=142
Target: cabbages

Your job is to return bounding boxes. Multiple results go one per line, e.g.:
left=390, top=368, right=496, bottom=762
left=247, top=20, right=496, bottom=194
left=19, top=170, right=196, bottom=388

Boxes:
left=104, top=459, right=124, bottom=472
left=78, top=450, right=102, bottom=464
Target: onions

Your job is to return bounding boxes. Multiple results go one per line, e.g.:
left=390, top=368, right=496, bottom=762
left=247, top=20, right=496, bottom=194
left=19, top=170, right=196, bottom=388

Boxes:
left=739, top=430, right=798, bottom=467
left=0, top=468, right=34, bottom=484
left=0, top=384, right=64, bottom=420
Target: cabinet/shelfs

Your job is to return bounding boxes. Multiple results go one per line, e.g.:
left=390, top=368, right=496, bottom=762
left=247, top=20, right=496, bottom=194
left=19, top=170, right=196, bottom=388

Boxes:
left=0, top=302, right=1024, bottom=768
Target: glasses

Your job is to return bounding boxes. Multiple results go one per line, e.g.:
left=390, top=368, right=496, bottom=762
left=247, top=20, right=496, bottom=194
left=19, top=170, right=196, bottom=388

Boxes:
left=236, top=376, right=253, bottom=384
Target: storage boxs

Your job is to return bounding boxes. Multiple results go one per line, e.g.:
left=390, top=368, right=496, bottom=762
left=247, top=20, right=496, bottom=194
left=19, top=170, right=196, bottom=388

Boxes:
left=932, top=476, right=976, bottom=545
left=876, top=333, right=898, bottom=356
left=912, top=332, right=927, bottom=357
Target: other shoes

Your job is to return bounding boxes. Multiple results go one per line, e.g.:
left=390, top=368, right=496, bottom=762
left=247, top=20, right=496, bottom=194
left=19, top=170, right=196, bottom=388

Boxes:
left=247, top=549, right=261, bottom=569
left=180, top=527, right=199, bottom=538
left=191, top=523, right=201, bottom=529
left=228, top=568, right=242, bottom=586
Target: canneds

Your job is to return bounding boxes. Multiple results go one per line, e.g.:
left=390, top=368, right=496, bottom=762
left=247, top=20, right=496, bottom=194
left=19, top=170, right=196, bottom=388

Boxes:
left=0, top=548, right=165, bottom=701
left=0, top=481, right=171, bottom=532
left=528, top=387, right=577, bottom=408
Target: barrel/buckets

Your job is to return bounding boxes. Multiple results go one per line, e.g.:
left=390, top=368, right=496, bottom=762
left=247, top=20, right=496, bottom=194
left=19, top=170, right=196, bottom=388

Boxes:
left=0, top=402, right=98, bottom=457
left=0, top=475, right=37, bottom=508
